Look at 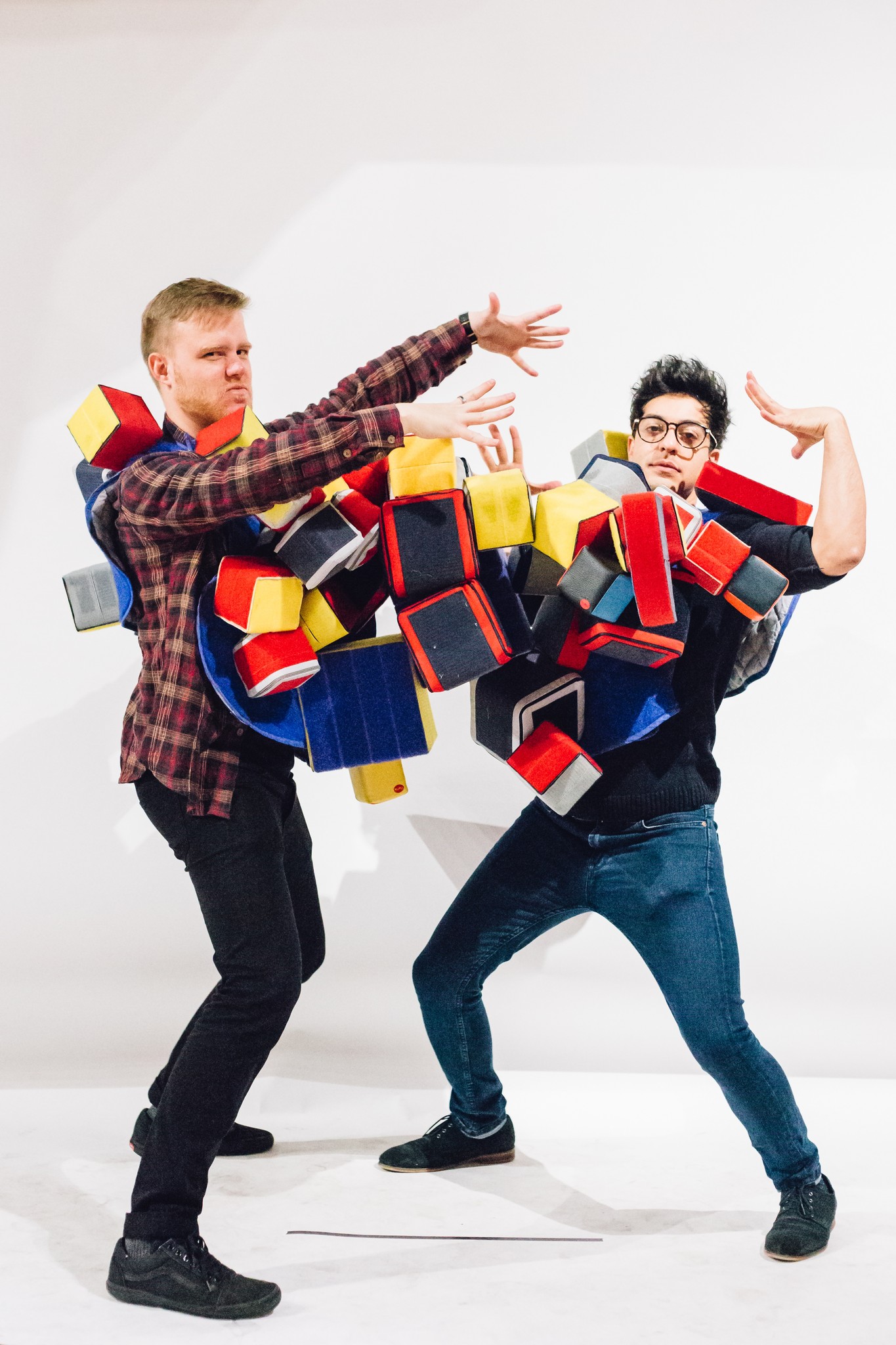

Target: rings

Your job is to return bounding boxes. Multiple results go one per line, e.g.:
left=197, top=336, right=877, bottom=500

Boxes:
left=457, top=396, right=466, bottom=402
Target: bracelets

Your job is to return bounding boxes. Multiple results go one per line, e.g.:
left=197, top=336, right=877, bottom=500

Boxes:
left=458, top=312, right=478, bottom=345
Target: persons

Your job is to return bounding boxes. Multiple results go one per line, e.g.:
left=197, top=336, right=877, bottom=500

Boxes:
left=83, top=277, right=571, bottom=1321
left=380, top=356, right=868, bottom=1262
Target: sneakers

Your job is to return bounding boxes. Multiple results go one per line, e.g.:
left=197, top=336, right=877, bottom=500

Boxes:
left=765, top=1173, right=837, bottom=1263
left=131, top=1106, right=274, bottom=1157
left=106, top=1227, right=281, bottom=1319
left=377, top=1107, right=515, bottom=1172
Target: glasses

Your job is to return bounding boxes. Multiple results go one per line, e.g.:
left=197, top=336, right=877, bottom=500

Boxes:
left=632, top=415, right=719, bottom=450
